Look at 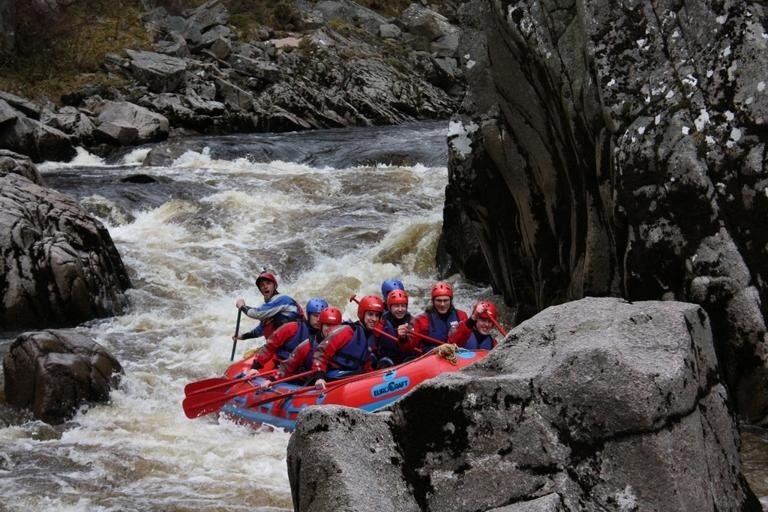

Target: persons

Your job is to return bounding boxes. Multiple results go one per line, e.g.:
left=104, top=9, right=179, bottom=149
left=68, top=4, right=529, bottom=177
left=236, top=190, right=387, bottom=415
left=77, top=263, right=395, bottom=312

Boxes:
left=233, top=271, right=306, bottom=343
left=311, top=294, right=384, bottom=391
left=374, top=279, right=499, bottom=366
left=260, top=307, right=342, bottom=391
left=246, top=298, right=328, bottom=377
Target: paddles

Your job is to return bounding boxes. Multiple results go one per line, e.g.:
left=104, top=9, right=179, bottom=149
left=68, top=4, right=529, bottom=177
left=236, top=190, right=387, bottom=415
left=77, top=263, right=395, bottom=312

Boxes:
left=183, top=365, right=382, bottom=420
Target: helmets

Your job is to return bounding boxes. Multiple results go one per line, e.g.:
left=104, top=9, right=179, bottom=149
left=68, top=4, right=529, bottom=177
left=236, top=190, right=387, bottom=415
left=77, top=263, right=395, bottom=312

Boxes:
left=319, top=306, right=342, bottom=325
left=256, top=273, right=277, bottom=287
left=472, top=301, right=497, bottom=321
left=358, top=279, right=408, bottom=321
left=431, top=284, right=453, bottom=301
left=306, top=297, right=328, bottom=315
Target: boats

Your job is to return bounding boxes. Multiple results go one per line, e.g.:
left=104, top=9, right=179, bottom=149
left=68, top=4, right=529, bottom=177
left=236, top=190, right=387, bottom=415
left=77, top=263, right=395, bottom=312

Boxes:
left=221, top=344, right=491, bottom=433
